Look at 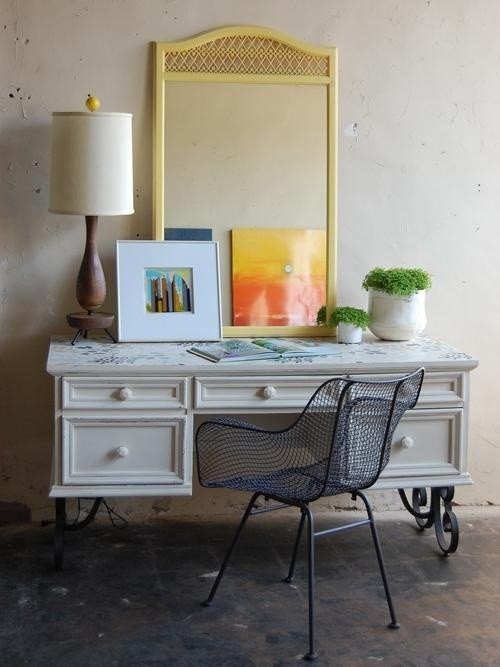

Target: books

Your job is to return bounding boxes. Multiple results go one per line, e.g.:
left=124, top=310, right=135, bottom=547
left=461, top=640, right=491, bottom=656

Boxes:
left=186, top=338, right=340, bottom=363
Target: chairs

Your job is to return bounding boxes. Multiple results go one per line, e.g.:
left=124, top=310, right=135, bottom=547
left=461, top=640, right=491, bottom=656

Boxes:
left=190, top=361, right=427, bottom=663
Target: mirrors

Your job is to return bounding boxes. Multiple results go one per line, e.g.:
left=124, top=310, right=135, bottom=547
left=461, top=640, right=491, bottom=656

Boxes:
left=150, top=22, right=336, bottom=340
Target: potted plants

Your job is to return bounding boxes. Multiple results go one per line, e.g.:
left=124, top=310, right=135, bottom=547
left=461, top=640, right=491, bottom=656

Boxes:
left=361, top=265, right=430, bottom=341
left=331, top=304, right=367, bottom=343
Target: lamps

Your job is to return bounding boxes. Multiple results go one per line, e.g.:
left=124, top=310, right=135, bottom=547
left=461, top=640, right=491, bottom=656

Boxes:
left=43, top=91, right=133, bottom=346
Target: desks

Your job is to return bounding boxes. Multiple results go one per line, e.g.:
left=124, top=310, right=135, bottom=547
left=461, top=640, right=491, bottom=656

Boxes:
left=42, top=331, right=477, bottom=555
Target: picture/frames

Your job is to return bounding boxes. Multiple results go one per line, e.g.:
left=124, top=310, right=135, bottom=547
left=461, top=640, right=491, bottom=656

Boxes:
left=111, top=236, right=223, bottom=344
